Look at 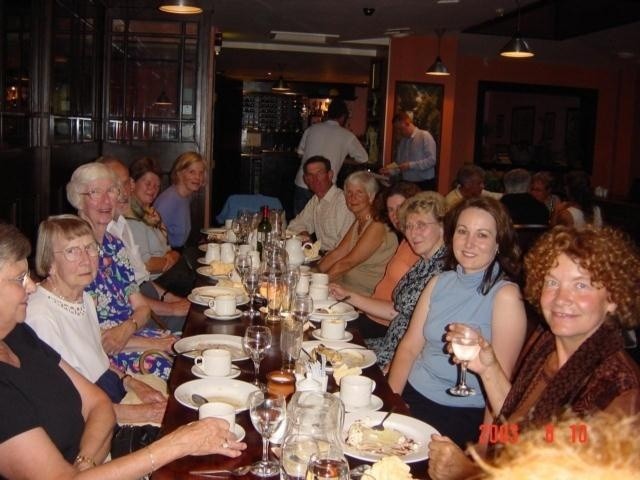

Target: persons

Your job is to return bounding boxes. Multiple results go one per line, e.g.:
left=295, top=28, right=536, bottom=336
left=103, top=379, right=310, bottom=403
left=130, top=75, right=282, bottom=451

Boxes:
left=306, top=109, right=322, bottom=126
left=380, top=108, right=437, bottom=190
left=1, top=155, right=640, bottom=480
left=292, top=97, right=369, bottom=217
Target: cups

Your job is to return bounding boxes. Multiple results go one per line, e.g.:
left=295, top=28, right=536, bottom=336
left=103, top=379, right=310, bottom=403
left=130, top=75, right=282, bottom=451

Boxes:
left=174, top=203, right=444, bottom=480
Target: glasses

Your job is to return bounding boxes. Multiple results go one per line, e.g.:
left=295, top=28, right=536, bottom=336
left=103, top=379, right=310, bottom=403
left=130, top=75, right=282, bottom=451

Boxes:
left=404, top=221, right=440, bottom=231
left=78, top=186, right=121, bottom=201
left=2, top=269, right=31, bottom=284
left=53, top=242, right=100, bottom=262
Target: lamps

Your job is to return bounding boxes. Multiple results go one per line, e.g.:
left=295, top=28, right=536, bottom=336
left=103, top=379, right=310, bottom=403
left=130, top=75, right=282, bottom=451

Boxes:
left=498, top=7, right=534, bottom=59
left=284, top=82, right=303, bottom=97
left=423, top=26, right=452, bottom=76
left=155, top=65, right=171, bottom=106
left=157, top=0, right=205, bottom=16
left=268, top=63, right=290, bottom=95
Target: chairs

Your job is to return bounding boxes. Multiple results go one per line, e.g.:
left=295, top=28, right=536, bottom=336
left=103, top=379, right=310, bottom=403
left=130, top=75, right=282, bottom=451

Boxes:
left=218, top=191, right=284, bottom=225
left=513, top=220, right=556, bottom=253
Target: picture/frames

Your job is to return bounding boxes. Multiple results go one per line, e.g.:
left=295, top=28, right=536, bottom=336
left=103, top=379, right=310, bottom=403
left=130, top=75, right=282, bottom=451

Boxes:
left=390, top=77, right=445, bottom=192
left=472, top=77, right=600, bottom=176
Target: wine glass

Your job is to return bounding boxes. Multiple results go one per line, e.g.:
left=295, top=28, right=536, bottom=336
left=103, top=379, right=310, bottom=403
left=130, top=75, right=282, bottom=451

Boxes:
left=446, top=324, right=481, bottom=398
